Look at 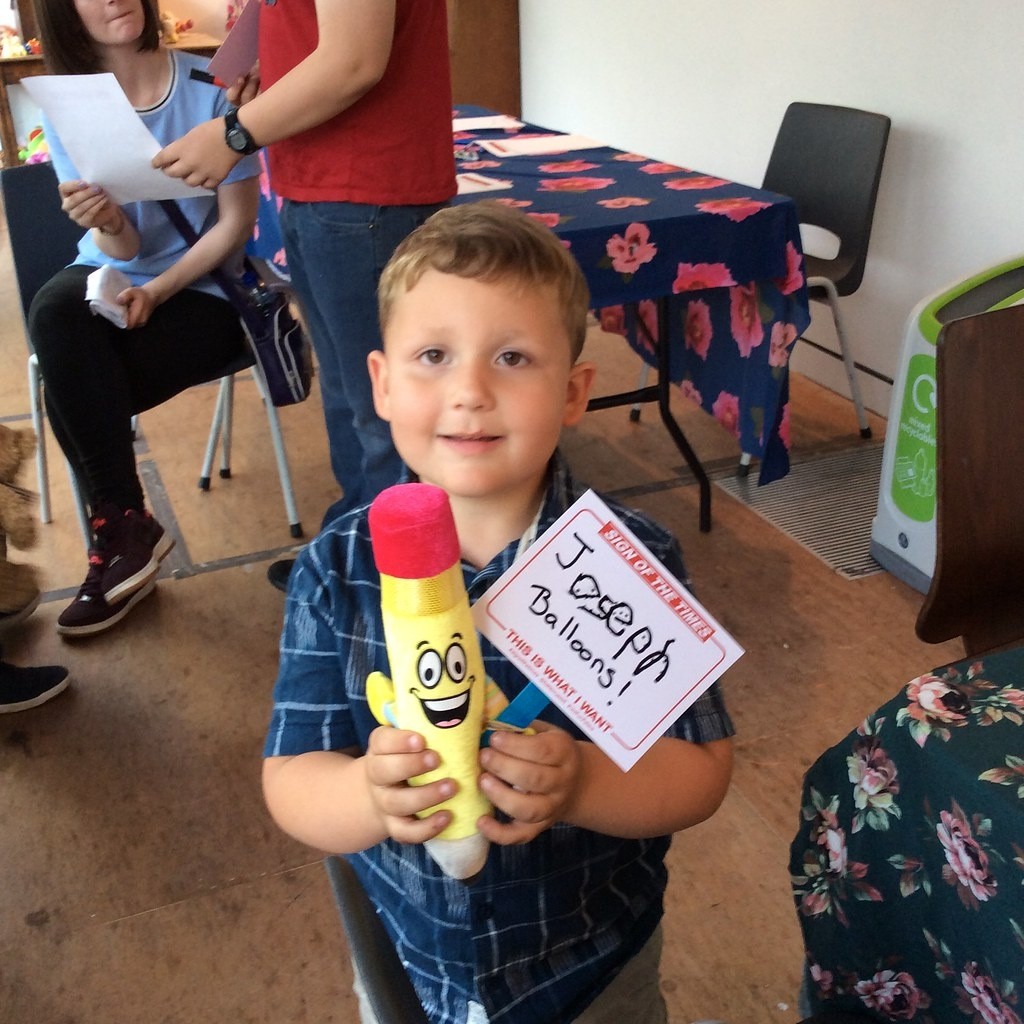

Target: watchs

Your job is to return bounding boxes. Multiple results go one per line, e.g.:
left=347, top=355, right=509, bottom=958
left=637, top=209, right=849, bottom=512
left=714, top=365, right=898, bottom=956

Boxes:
left=224, top=108, right=262, bottom=154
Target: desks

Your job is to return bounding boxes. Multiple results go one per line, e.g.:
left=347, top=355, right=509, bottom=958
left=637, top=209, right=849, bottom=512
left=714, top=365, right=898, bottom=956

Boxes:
left=448, top=103, right=813, bottom=534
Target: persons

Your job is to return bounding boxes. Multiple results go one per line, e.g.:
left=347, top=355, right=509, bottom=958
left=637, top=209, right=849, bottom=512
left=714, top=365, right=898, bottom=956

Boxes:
left=0, top=553, right=69, bottom=715
left=262, top=201, right=737, bottom=1024
left=27, top=0, right=259, bottom=633
left=152, top=0, right=458, bottom=596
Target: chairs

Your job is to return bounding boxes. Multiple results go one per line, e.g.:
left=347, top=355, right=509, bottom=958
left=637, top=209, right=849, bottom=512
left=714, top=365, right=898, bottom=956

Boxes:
left=630, top=102, right=892, bottom=479
left=912, top=303, right=1024, bottom=656
left=28, top=354, right=303, bottom=552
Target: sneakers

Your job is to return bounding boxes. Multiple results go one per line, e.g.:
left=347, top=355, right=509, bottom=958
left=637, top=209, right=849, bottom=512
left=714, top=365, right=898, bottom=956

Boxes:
left=57, top=510, right=176, bottom=637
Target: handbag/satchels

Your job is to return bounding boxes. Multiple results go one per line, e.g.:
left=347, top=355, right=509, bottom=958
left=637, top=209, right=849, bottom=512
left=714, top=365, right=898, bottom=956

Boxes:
left=239, top=283, right=312, bottom=408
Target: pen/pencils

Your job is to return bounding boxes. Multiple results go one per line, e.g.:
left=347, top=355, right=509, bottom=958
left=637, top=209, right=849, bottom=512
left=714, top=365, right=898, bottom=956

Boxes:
left=458, top=142, right=474, bottom=156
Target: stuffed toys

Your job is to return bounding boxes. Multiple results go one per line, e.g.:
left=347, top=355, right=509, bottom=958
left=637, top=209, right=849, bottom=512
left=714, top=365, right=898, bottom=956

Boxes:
left=0, top=425, right=37, bottom=613
left=366, top=483, right=533, bottom=881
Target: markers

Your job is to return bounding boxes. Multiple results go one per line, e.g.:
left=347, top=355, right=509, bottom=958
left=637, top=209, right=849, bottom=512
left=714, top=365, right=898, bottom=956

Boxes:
left=190, top=67, right=230, bottom=89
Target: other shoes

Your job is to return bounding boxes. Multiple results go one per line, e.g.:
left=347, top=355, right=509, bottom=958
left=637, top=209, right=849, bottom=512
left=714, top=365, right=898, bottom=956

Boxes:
left=0, top=585, right=40, bottom=630
left=268, top=559, right=296, bottom=590
left=1, top=662, right=70, bottom=713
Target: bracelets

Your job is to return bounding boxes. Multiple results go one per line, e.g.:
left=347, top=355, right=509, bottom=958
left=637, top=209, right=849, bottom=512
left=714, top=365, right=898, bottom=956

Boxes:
left=98, top=209, right=124, bottom=236
